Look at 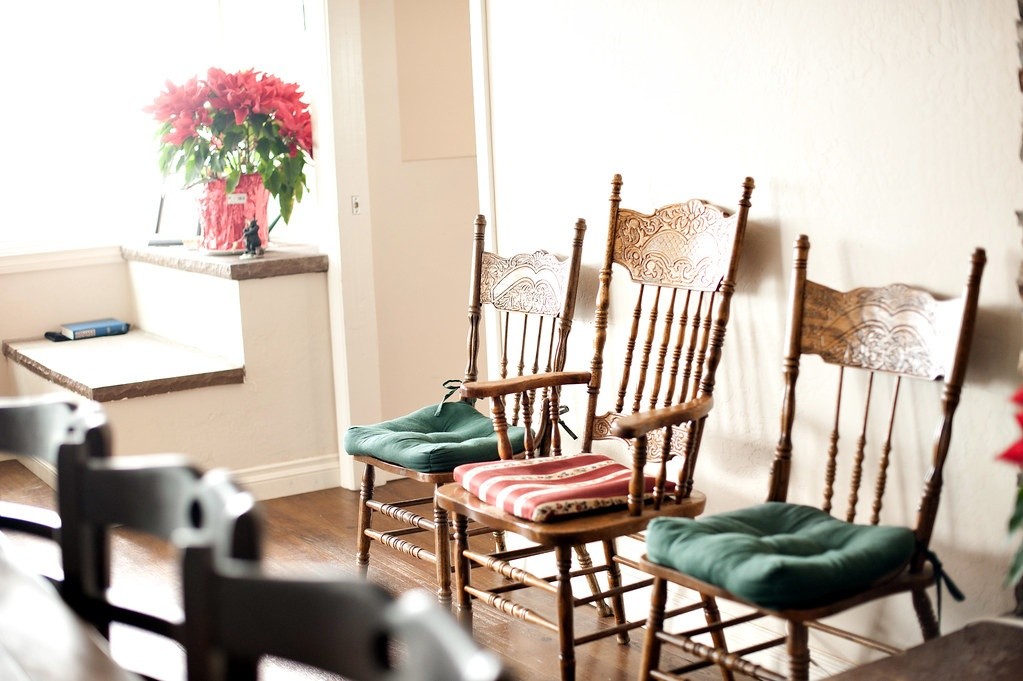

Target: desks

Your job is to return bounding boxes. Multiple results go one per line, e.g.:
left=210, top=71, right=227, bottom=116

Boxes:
left=0, top=556, right=146, bottom=681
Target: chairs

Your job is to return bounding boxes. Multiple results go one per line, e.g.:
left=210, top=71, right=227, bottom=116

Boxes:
left=0, top=389, right=515, bottom=681
left=639, top=234, right=992, bottom=681
left=344, top=214, right=586, bottom=613
left=435, top=174, right=755, bottom=681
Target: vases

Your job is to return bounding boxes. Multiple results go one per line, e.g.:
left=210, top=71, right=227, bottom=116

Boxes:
left=205, top=166, right=268, bottom=252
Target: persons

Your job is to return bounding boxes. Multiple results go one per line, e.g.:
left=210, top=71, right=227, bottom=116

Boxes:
left=243, top=221, right=262, bottom=254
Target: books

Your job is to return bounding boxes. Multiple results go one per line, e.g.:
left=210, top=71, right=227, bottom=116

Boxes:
left=61, top=318, right=128, bottom=340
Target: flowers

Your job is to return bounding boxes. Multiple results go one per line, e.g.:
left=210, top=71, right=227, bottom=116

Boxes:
left=157, top=64, right=310, bottom=226
